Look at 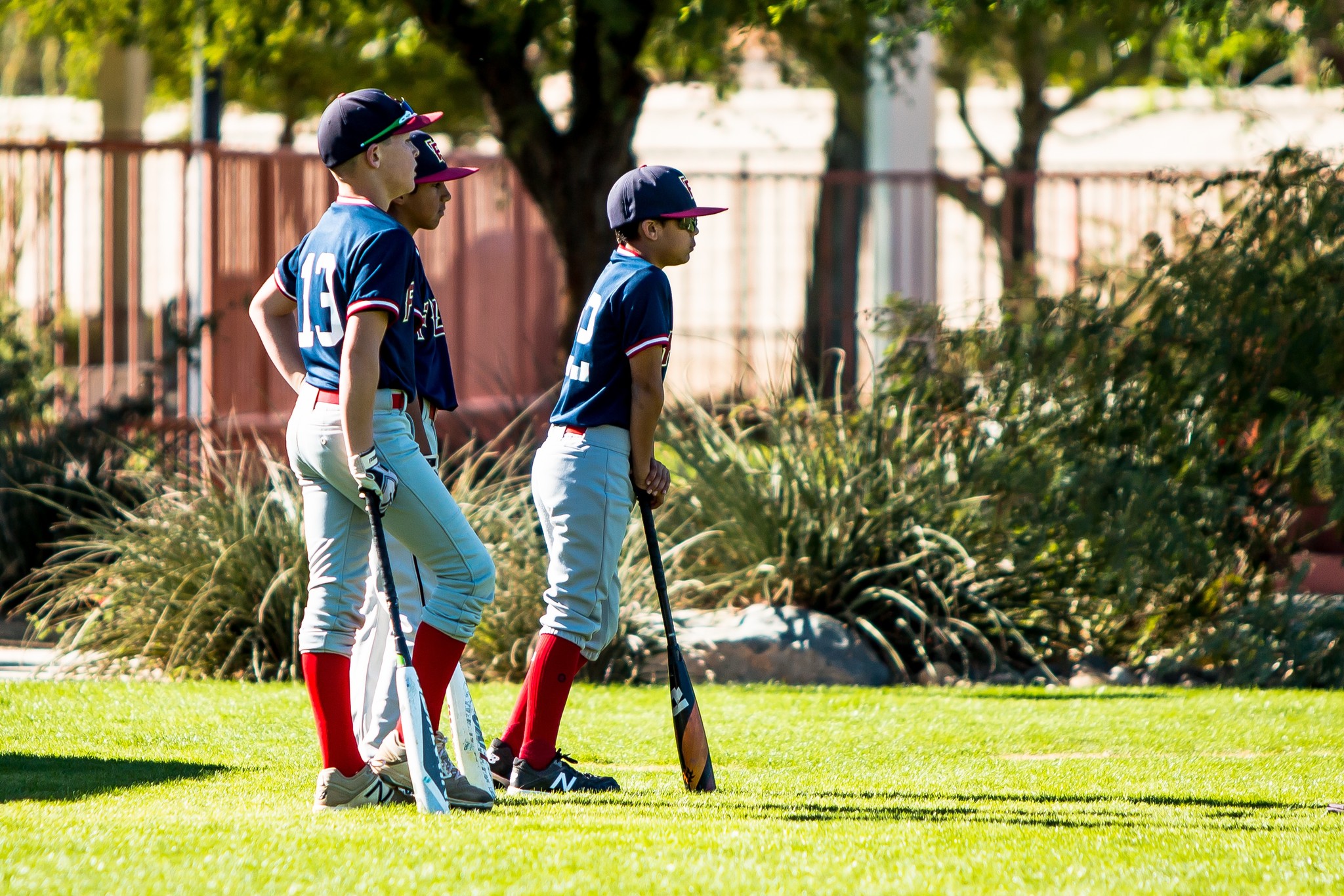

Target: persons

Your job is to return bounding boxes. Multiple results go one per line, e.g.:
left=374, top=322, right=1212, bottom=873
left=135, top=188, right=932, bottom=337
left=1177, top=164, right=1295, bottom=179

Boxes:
left=489, top=164, right=730, bottom=792
left=247, top=89, right=502, bottom=811
left=352, top=127, right=484, bottom=760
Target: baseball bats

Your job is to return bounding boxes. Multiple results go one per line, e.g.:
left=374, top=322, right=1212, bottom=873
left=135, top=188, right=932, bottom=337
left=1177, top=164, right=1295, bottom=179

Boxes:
left=360, top=483, right=451, bottom=816
left=444, top=658, right=498, bottom=802
left=629, top=470, right=718, bottom=794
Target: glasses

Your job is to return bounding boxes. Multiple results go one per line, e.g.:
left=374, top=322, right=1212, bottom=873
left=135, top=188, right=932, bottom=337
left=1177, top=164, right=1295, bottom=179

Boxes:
left=360, top=100, right=418, bottom=147
left=678, top=218, right=699, bottom=233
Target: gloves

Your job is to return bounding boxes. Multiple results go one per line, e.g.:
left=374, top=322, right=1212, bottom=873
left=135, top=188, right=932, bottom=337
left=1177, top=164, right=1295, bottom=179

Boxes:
left=345, top=445, right=400, bottom=520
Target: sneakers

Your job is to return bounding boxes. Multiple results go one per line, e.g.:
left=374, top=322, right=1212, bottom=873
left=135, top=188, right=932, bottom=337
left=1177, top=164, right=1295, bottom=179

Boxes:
left=508, top=754, right=620, bottom=798
left=371, top=729, right=495, bottom=811
left=482, top=737, right=509, bottom=791
left=316, top=763, right=389, bottom=808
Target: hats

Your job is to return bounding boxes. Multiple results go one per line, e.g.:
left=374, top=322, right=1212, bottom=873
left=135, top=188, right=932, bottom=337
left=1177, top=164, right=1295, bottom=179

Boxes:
left=413, top=128, right=480, bottom=185
left=317, top=89, right=444, bottom=173
left=606, top=165, right=728, bottom=228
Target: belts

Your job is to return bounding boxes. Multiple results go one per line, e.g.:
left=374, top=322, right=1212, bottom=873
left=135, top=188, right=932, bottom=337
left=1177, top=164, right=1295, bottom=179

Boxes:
left=565, top=423, right=587, bottom=435
left=317, top=388, right=405, bottom=409
left=418, top=398, right=439, bottom=422
